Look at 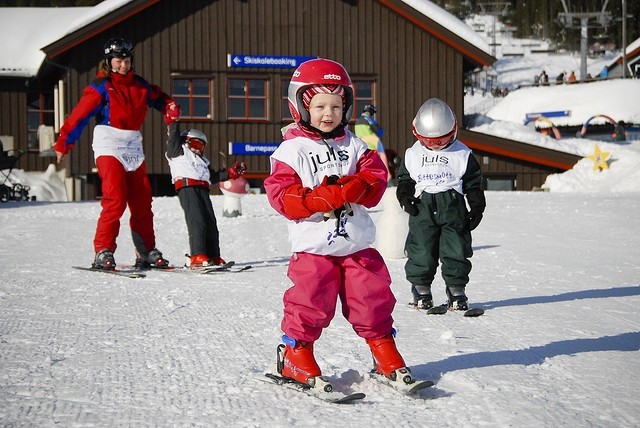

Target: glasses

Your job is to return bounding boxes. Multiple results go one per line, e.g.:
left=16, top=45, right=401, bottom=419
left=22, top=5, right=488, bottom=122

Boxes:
left=412, top=121, right=457, bottom=146
left=106, top=40, right=133, bottom=53
left=186, top=137, right=206, bottom=153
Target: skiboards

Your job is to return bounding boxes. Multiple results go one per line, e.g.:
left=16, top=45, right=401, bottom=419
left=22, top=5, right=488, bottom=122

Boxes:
left=175, top=261, right=253, bottom=272
left=407, top=299, right=484, bottom=317
left=253, top=371, right=435, bottom=403
left=71, top=264, right=217, bottom=278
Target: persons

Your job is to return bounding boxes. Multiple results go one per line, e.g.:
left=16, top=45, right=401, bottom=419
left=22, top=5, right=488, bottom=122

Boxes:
left=264, top=59, right=406, bottom=384
left=54, top=39, right=181, bottom=269
left=166, top=102, right=247, bottom=268
left=556, top=73, right=564, bottom=84
left=569, top=71, right=576, bottom=84
left=540, top=71, right=549, bottom=86
left=355, top=104, right=395, bottom=187
left=396, top=99, right=486, bottom=309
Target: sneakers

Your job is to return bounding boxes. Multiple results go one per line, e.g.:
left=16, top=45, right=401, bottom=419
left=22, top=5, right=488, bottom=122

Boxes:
left=281, top=339, right=321, bottom=385
left=412, top=285, right=433, bottom=309
left=209, top=256, right=225, bottom=265
left=190, top=254, right=214, bottom=266
left=92, top=248, right=116, bottom=270
left=366, top=334, right=406, bottom=380
left=136, top=249, right=169, bottom=268
left=446, top=286, right=468, bottom=310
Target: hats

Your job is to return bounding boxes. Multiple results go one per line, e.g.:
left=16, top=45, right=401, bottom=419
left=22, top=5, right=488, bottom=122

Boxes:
left=303, top=85, right=345, bottom=110
left=363, top=105, right=376, bottom=113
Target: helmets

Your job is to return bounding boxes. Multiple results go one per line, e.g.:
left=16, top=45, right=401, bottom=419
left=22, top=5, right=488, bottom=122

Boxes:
left=412, top=98, right=457, bottom=138
left=288, top=59, right=355, bottom=125
left=105, top=38, right=135, bottom=72
left=180, top=128, right=208, bottom=145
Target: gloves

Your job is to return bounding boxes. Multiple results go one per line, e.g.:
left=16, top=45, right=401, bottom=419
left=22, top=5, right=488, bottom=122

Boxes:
left=466, top=187, right=486, bottom=231
left=228, top=161, right=247, bottom=180
left=283, top=176, right=344, bottom=219
left=396, top=179, right=420, bottom=217
left=336, top=171, right=386, bottom=210
left=164, top=100, right=181, bottom=125
